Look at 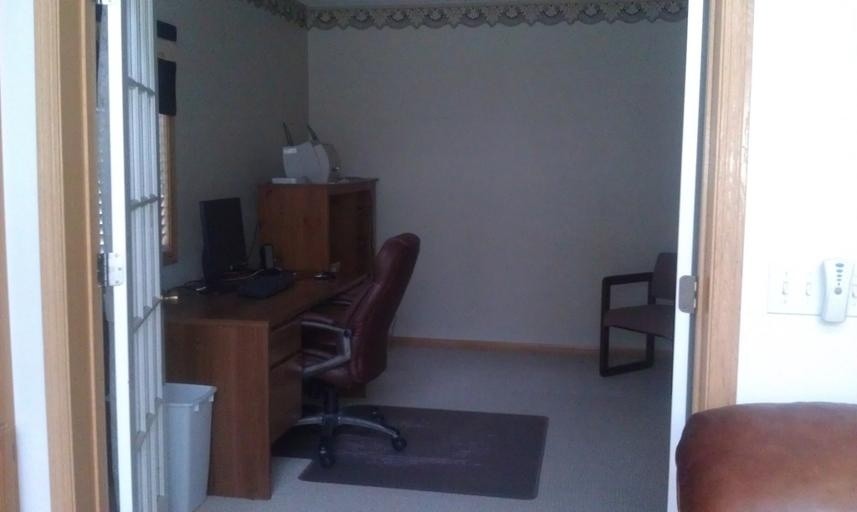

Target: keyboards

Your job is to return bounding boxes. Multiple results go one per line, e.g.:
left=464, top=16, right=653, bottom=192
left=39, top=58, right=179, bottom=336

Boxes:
left=239, top=275, right=289, bottom=298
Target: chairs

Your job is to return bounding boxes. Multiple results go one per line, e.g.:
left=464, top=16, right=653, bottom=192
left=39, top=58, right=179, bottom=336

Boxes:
left=597, top=252, right=674, bottom=380
left=280, top=231, right=421, bottom=468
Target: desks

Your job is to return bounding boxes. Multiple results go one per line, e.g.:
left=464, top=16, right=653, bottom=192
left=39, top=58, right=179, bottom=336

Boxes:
left=162, top=272, right=369, bottom=501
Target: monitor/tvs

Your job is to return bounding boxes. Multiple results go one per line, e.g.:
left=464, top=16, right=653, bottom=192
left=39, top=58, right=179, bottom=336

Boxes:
left=199, top=198, right=249, bottom=291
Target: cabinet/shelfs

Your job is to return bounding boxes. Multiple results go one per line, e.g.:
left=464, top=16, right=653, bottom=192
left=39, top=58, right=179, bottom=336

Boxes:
left=262, top=180, right=377, bottom=272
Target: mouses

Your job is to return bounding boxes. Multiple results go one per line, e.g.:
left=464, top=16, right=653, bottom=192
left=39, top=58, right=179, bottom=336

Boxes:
left=313, top=272, right=335, bottom=279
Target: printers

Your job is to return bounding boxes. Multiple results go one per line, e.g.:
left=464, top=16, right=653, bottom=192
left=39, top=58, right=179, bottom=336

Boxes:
left=280, top=122, right=341, bottom=183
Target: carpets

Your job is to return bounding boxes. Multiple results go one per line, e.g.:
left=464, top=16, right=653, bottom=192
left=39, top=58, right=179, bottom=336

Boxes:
left=297, top=403, right=551, bottom=499
